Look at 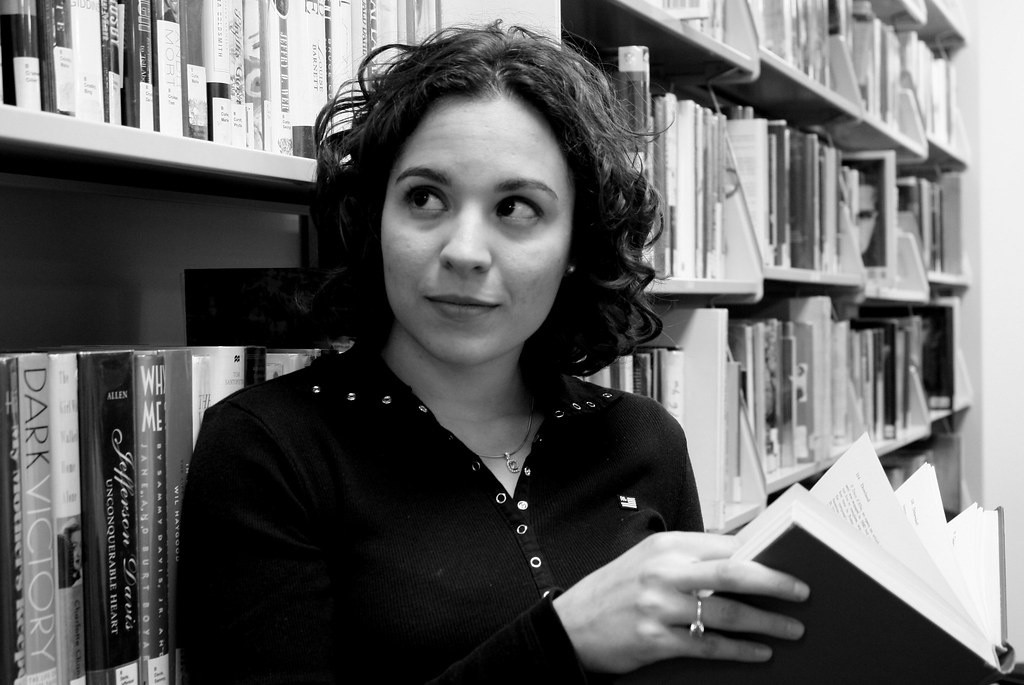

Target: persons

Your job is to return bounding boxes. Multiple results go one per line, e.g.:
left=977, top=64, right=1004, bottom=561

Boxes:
left=176, top=29, right=812, bottom=685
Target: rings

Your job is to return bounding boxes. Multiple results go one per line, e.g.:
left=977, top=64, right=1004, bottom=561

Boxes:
left=689, top=595, right=705, bottom=640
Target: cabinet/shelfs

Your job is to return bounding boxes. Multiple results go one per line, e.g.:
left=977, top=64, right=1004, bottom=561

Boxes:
left=0, top=0, right=978, bottom=685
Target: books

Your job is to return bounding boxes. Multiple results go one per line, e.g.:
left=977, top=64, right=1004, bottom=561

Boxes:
left=638, top=431, right=1016, bottom=685
left=0, top=339, right=351, bottom=685
left=0, top=0, right=445, bottom=158
left=602, top=45, right=968, bottom=516
left=647, top=0, right=973, bottom=159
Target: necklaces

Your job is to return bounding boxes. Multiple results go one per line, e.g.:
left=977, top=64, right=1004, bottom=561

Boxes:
left=474, top=393, right=536, bottom=473
